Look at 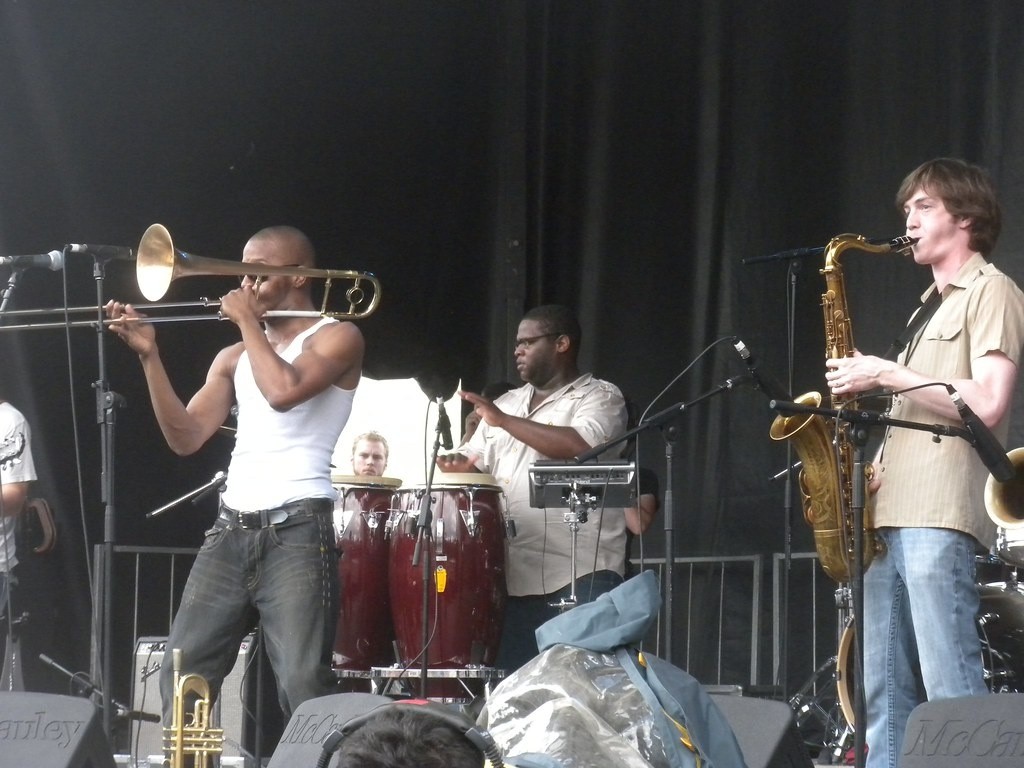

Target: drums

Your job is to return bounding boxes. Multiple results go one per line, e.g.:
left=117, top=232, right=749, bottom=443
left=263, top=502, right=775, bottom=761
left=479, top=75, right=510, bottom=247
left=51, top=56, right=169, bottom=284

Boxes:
left=975, top=554, right=1002, bottom=585
left=995, top=526, right=1024, bottom=568
left=329, top=474, right=510, bottom=706
left=835, top=579, right=1024, bottom=737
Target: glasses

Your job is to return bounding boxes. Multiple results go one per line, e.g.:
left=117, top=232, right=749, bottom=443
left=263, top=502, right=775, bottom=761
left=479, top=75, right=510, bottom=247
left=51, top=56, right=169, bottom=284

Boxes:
left=513, top=331, right=568, bottom=350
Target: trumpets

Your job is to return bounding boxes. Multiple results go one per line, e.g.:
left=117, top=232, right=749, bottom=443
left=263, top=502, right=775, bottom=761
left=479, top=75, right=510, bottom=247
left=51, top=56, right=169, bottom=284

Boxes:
left=161, top=646, right=226, bottom=768
left=983, top=446, right=1024, bottom=530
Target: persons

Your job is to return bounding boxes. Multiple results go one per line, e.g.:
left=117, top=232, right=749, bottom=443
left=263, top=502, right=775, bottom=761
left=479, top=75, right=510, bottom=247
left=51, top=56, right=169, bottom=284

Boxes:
left=459, top=382, right=516, bottom=450
left=352, top=432, right=390, bottom=478
left=336, top=700, right=485, bottom=768
left=624, top=468, right=660, bottom=579
left=106, top=225, right=364, bottom=768
left=823, top=156, right=1024, bottom=768
left=431, top=304, right=629, bottom=686
left=0, top=400, right=38, bottom=679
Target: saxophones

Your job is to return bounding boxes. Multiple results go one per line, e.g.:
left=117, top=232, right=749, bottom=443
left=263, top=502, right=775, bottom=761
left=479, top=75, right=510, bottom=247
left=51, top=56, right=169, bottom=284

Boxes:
left=766, top=229, right=921, bottom=584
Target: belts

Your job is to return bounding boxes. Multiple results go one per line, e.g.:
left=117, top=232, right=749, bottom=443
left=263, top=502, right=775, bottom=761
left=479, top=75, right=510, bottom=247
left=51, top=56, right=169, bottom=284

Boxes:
left=219, top=496, right=334, bottom=529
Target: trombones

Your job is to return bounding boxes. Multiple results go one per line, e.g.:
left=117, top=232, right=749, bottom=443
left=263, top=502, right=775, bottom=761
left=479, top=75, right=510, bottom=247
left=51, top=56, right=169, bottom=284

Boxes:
left=0, top=222, right=385, bottom=334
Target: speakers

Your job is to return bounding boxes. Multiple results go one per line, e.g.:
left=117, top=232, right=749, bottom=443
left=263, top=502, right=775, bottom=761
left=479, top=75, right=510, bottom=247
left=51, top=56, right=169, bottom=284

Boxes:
left=265, top=692, right=394, bottom=768
left=0, top=690, right=118, bottom=768
left=707, top=693, right=815, bottom=768
left=128, top=627, right=284, bottom=761
left=898, top=692, right=1024, bottom=768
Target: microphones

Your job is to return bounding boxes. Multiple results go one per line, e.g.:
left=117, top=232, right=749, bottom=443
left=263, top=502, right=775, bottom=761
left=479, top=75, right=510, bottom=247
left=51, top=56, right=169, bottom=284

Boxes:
left=0, top=250, right=63, bottom=272
left=732, top=336, right=797, bottom=417
left=946, top=384, right=1016, bottom=481
left=66, top=244, right=138, bottom=260
left=437, top=392, right=453, bottom=450
left=116, top=708, right=160, bottom=723
